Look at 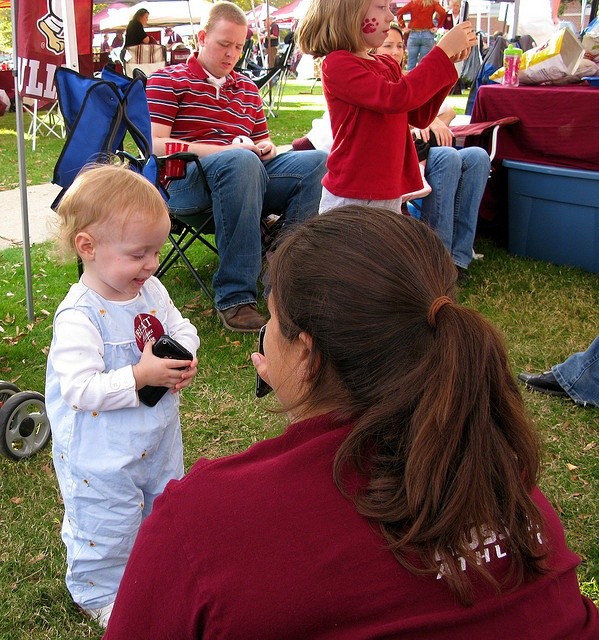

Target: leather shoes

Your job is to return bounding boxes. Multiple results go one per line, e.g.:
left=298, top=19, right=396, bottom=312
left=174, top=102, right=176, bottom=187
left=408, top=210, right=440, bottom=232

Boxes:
left=218, top=304, right=268, bottom=332
left=518, top=371, right=568, bottom=396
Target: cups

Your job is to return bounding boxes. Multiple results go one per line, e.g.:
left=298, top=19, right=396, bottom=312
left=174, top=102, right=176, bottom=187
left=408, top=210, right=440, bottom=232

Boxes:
left=503, top=46, right=523, bottom=87
left=165, top=142, right=189, bottom=178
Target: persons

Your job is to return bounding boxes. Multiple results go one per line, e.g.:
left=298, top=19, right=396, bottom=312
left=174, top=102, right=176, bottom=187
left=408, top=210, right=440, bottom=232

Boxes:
left=123, top=8, right=159, bottom=51
left=99, top=34, right=112, bottom=53
left=398, top=3, right=445, bottom=73
left=145, top=2, right=330, bottom=334
left=368, top=24, right=489, bottom=278
left=293, top=0, right=479, bottom=214
left=515, top=335, right=598, bottom=404
left=165, top=26, right=184, bottom=51
left=43, top=164, right=201, bottom=630
left=104, top=204, right=599, bottom=634
left=114, top=28, right=122, bottom=51
left=264, top=15, right=281, bottom=69
left=445, top=0, right=468, bottom=27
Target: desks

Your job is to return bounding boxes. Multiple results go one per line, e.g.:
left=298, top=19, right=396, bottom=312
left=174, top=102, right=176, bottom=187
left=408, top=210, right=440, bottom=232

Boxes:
left=461, top=83, right=598, bottom=172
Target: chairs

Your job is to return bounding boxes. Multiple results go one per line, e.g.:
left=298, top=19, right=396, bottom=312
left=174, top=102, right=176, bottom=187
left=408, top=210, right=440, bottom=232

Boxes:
left=50, top=63, right=270, bottom=315
left=316, top=114, right=520, bottom=259
left=233, top=36, right=254, bottom=72
left=250, top=32, right=295, bottom=119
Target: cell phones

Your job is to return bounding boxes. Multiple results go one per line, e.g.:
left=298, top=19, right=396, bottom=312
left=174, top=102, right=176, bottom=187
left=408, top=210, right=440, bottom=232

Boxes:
left=458, top=0, right=469, bottom=25
left=252, top=148, right=271, bottom=155
left=137, top=335, right=194, bottom=408
left=255, top=323, right=273, bottom=398
